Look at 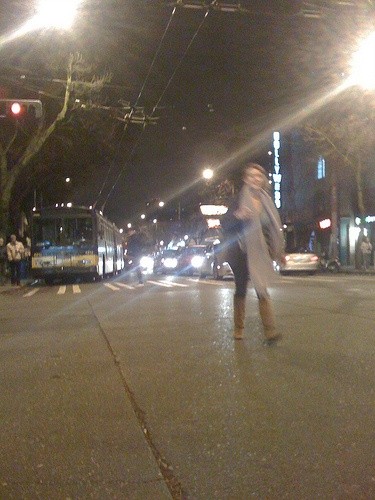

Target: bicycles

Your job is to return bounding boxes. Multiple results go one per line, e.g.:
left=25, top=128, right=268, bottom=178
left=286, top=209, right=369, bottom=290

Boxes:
left=320, top=253, right=340, bottom=273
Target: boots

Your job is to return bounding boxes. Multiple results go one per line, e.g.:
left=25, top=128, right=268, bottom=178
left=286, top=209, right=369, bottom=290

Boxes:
left=233, top=294, right=246, bottom=339
left=257, top=295, right=282, bottom=341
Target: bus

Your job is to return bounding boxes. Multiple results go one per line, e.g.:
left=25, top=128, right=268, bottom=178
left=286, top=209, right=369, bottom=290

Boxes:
left=30, top=206, right=123, bottom=283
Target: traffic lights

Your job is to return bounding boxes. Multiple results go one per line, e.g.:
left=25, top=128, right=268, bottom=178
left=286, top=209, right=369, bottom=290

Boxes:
left=0, top=100, right=43, bottom=119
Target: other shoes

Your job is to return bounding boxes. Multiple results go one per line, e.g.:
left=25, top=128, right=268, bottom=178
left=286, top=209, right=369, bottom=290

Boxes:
left=11, top=281, right=16, bottom=285
left=17, top=282, right=22, bottom=287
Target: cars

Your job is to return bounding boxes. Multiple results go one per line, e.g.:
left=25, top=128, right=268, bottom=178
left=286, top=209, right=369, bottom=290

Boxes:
left=279, top=250, right=318, bottom=275
left=200, top=240, right=234, bottom=279
left=181, top=245, right=207, bottom=276
left=153, top=249, right=181, bottom=273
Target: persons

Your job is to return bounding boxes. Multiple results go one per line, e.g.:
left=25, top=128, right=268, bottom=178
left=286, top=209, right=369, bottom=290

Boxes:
left=360, top=237, right=373, bottom=273
left=126, top=227, right=144, bottom=288
left=0, top=234, right=26, bottom=287
left=219, top=163, right=283, bottom=342
